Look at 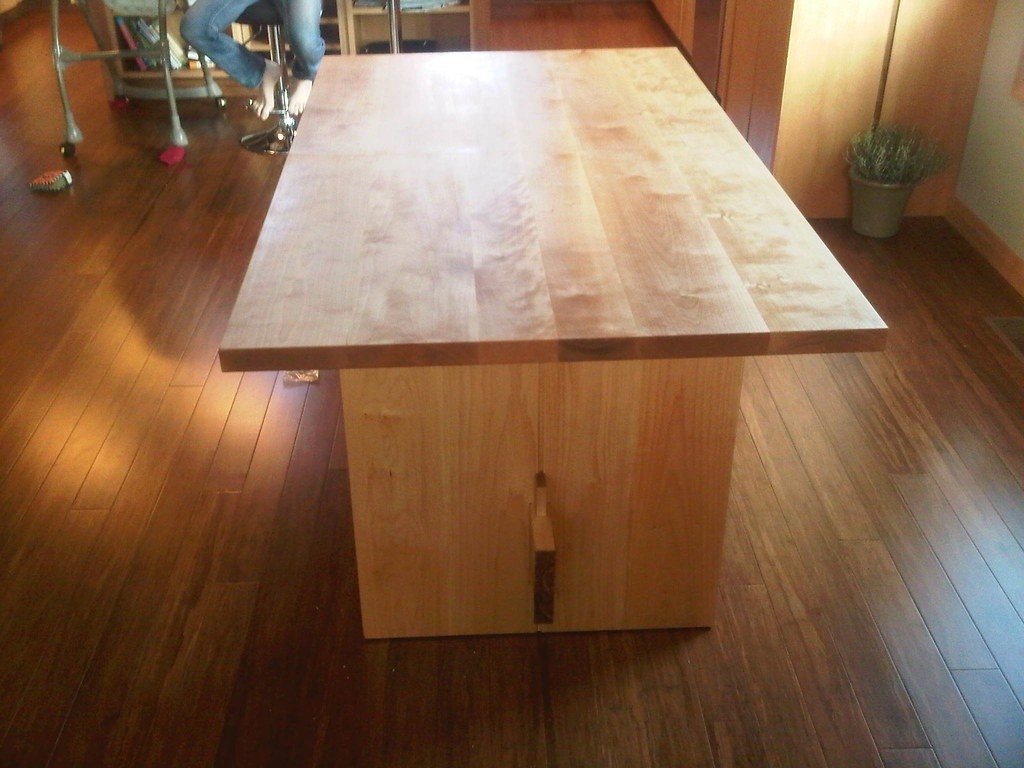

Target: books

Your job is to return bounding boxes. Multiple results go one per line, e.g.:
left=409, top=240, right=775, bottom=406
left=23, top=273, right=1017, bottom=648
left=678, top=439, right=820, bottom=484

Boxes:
left=116, top=17, right=186, bottom=70
left=188, top=50, right=216, bottom=70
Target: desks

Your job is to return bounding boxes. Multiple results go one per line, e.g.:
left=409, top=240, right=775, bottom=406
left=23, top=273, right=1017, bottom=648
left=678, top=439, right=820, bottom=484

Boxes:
left=219, top=0, right=889, bottom=642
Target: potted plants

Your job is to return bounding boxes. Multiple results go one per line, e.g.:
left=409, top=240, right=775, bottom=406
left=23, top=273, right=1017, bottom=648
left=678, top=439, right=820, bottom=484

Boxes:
left=845, top=118, right=953, bottom=238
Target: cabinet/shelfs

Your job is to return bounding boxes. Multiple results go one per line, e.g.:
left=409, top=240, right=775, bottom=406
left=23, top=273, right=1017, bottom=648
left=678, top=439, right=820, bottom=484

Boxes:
left=96, top=0, right=470, bottom=103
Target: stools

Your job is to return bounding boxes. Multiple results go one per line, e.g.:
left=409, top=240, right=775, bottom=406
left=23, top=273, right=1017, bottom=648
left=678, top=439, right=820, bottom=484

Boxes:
left=234, top=0, right=337, bottom=155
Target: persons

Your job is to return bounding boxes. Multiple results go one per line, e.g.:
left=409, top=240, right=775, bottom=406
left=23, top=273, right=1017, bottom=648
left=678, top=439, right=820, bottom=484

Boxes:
left=180, top=0, right=324, bottom=121
